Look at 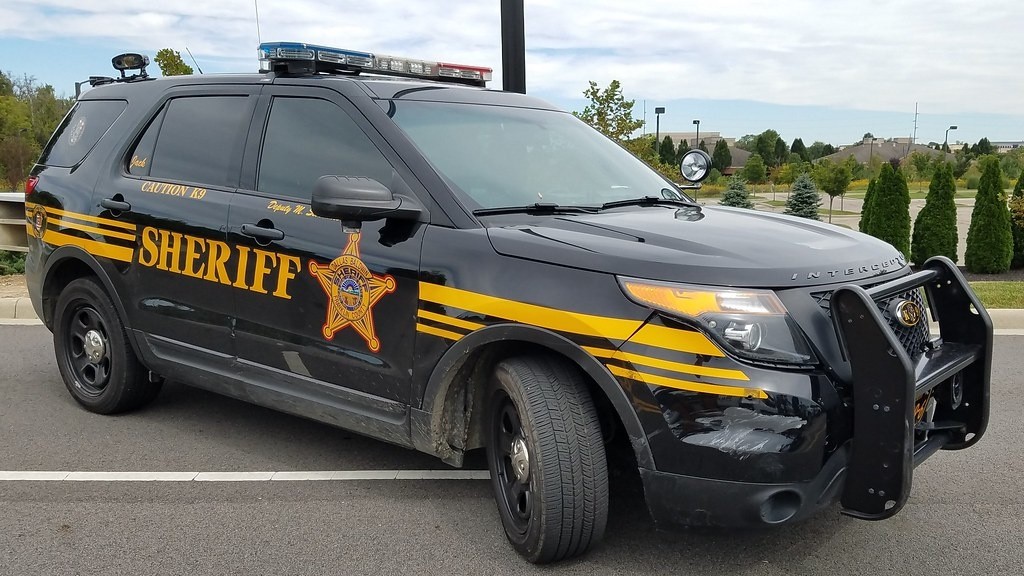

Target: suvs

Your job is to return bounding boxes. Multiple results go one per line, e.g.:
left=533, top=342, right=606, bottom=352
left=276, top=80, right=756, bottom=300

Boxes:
left=26, top=41, right=994, bottom=569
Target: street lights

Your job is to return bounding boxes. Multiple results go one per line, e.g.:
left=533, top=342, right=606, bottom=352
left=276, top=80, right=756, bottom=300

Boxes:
left=692, top=119, right=701, bottom=203
left=655, top=107, right=665, bottom=155
left=944, top=126, right=958, bottom=172
